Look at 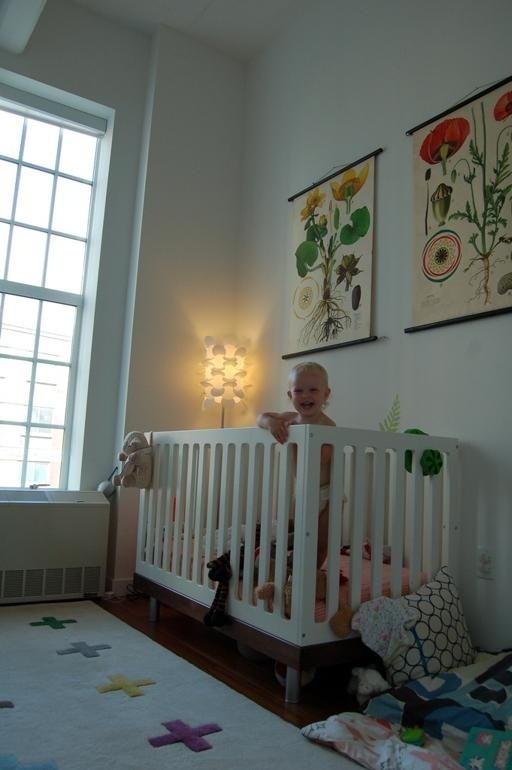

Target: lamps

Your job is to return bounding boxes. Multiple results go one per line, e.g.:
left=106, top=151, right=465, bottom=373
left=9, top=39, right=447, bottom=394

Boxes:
left=198, top=332, right=252, bottom=428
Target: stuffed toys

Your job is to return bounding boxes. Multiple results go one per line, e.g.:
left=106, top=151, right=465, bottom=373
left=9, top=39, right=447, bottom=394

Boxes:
left=203, top=523, right=352, bottom=638
left=112, top=430, right=152, bottom=488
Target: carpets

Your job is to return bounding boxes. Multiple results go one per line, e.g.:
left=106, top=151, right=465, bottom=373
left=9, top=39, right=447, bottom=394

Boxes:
left=0, top=600, right=365, bottom=769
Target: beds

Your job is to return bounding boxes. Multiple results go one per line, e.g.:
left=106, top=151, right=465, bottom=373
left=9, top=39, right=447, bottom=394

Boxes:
left=132, top=423, right=463, bottom=704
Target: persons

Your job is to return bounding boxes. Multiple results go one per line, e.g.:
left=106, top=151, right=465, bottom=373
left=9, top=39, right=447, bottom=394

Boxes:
left=258, top=362, right=347, bottom=570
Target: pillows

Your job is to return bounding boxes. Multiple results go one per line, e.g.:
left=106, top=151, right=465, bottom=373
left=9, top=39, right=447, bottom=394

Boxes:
left=387, top=566, right=476, bottom=688
